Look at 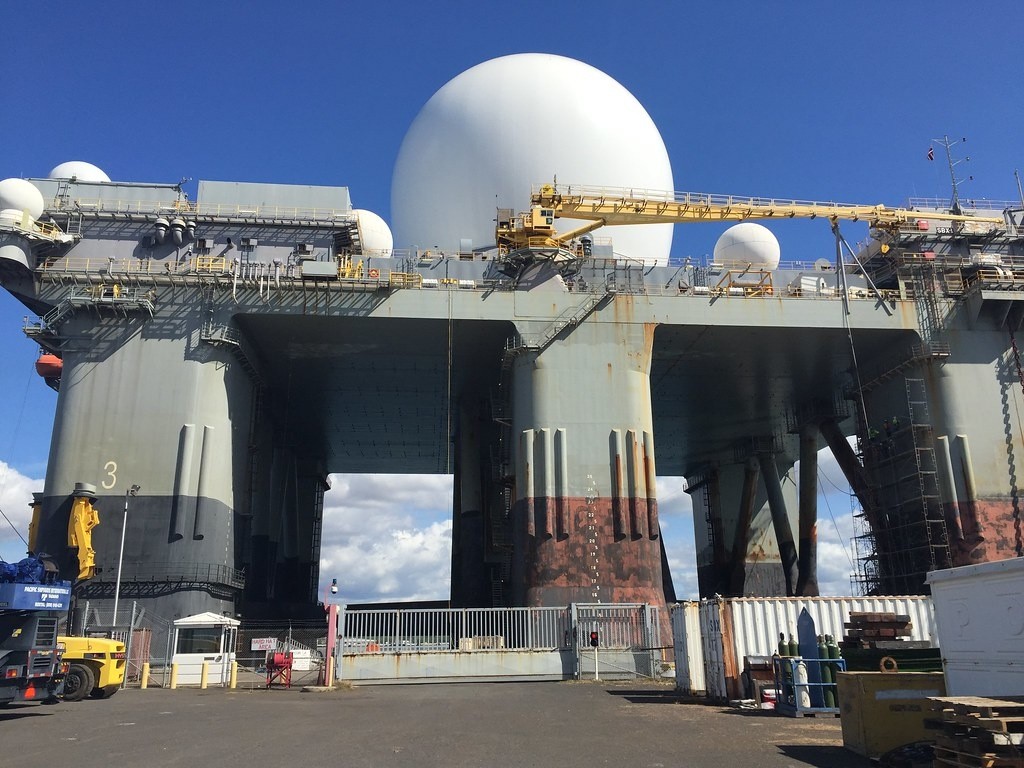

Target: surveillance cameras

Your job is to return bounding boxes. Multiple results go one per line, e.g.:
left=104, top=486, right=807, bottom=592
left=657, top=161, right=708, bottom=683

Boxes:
left=331, top=586, right=338, bottom=594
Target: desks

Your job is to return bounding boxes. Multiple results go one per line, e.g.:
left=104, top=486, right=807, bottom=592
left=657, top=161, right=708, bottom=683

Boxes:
left=751, top=679, right=775, bottom=707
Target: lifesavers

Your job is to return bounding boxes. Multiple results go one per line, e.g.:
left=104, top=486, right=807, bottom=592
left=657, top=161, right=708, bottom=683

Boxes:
left=369, top=269, right=379, bottom=277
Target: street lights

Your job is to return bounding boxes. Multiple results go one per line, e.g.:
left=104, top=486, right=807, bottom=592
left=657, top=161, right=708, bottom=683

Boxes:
left=113, top=484, right=141, bottom=628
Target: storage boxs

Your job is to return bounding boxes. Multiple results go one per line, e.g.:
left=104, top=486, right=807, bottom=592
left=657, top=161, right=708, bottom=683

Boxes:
left=836, top=670, right=947, bottom=760
left=744, top=655, right=773, bottom=679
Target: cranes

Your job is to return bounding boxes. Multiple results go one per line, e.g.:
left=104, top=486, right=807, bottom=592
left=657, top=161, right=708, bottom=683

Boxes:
left=494, top=174, right=1004, bottom=262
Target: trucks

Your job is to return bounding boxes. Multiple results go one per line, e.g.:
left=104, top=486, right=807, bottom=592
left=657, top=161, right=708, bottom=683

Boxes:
left=0, top=580, right=72, bottom=709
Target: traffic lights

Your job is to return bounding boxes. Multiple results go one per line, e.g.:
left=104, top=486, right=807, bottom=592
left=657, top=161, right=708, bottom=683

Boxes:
left=591, top=631, right=598, bottom=646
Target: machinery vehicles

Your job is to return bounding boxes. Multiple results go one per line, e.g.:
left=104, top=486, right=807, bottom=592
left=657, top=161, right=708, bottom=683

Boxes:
left=57, top=636, right=127, bottom=703
